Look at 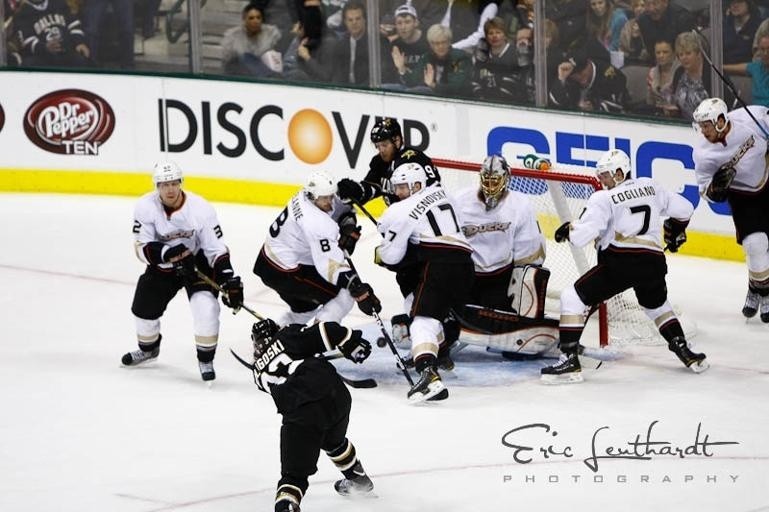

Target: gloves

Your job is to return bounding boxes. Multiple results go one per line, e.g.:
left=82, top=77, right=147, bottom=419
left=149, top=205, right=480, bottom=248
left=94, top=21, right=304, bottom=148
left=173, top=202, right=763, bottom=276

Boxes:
left=339, top=330, right=371, bottom=364
left=708, top=163, right=736, bottom=202
left=350, top=282, right=383, bottom=315
left=555, top=220, right=570, bottom=243
left=664, top=217, right=690, bottom=254
left=339, top=223, right=362, bottom=254
left=165, top=243, right=197, bottom=278
left=220, top=276, right=244, bottom=312
left=338, top=180, right=366, bottom=205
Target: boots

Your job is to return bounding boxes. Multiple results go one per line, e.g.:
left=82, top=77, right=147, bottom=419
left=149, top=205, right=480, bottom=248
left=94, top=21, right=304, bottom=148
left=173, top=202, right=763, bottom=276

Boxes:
left=122, top=333, right=161, bottom=366
left=541, top=344, right=582, bottom=372
left=336, top=467, right=375, bottom=495
left=667, top=337, right=706, bottom=366
left=197, top=348, right=217, bottom=380
left=397, top=337, right=458, bottom=397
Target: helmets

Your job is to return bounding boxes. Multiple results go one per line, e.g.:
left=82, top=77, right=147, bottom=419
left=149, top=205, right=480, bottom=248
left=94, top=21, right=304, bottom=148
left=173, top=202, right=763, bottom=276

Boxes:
left=306, top=173, right=338, bottom=199
left=390, top=163, right=427, bottom=193
left=370, top=119, right=402, bottom=142
left=481, top=155, right=511, bottom=207
left=693, top=97, right=731, bottom=126
left=595, top=150, right=630, bottom=189
left=155, top=160, right=182, bottom=187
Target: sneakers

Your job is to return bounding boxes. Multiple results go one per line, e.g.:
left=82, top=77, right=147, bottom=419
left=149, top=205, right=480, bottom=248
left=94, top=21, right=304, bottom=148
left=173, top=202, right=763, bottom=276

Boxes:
left=742, top=285, right=769, bottom=323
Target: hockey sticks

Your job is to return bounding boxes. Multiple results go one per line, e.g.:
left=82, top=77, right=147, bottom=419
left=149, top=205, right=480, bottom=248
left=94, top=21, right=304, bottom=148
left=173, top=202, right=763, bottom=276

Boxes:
left=698, top=39, right=769, bottom=137
left=194, top=266, right=377, bottom=389
left=574, top=305, right=621, bottom=363
left=502, top=338, right=560, bottom=361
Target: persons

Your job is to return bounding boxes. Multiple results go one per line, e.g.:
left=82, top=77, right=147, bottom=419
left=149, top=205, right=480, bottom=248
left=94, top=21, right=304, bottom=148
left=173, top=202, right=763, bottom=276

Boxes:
left=251, top=317, right=371, bottom=512
left=337, top=117, right=441, bottom=205
left=693, top=97, right=769, bottom=324
left=3, top=1, right=157, bottom=71
left=254, top=175, right=381, bottom=326
left=540, top=149, right=706, bottom=374
left=221, top=2, right=768, bottom=119
left=376, top=163, right=474, bottom=400
left=121, top=160, right=242, bottom=381
left=453, top=155, right=544, bottom=272
